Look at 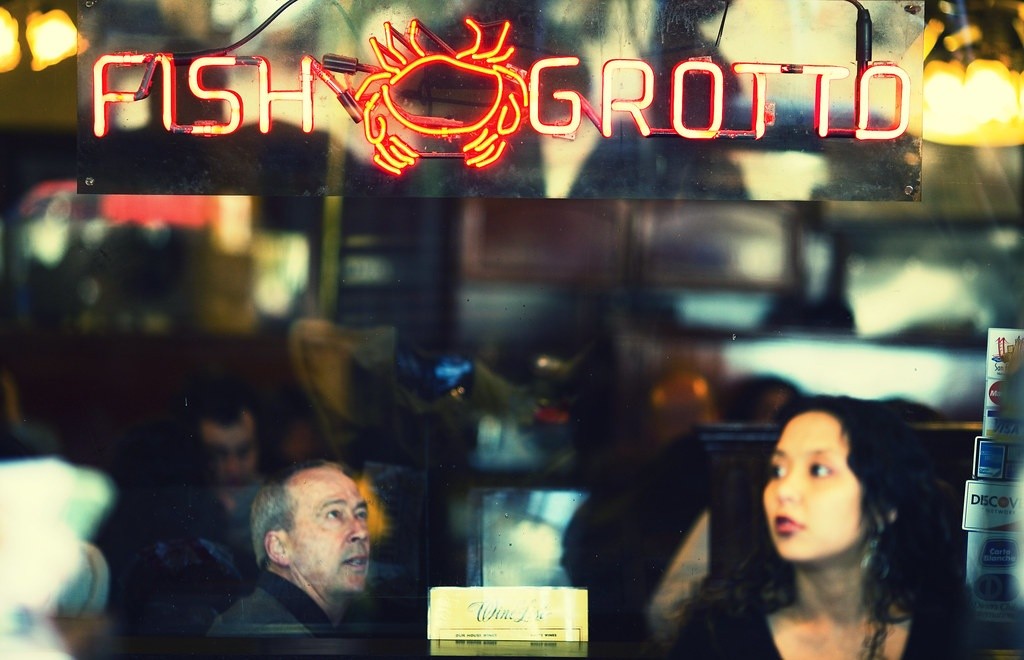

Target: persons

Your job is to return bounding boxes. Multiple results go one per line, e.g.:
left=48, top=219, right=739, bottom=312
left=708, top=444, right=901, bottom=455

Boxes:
left=666, top=396, right=985, bottom=660
left=211, top=459, right=376, bottom=638
